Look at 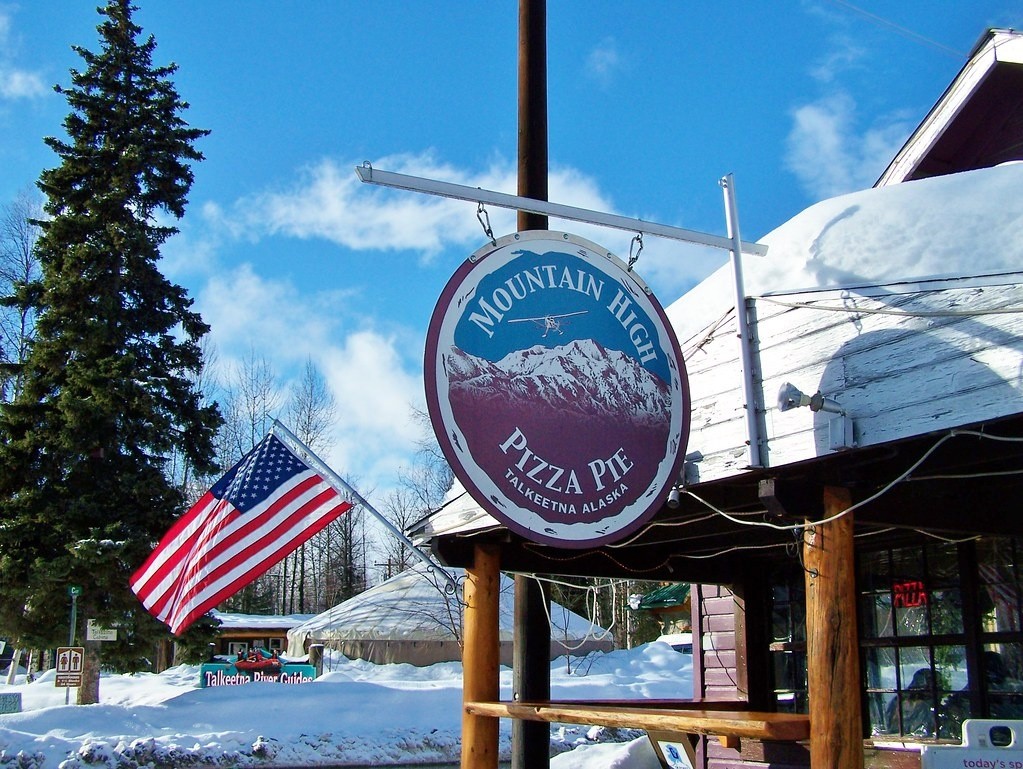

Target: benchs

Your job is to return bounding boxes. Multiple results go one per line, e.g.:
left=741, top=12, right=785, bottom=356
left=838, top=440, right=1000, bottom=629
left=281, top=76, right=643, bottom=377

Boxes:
left=461, top=699, right=810, bottom=743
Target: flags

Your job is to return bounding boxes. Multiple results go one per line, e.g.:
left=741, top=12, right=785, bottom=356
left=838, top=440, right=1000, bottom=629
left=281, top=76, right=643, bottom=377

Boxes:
left=128, top=428, right=353, bottom=636
left=978, top=562, right=1023, bottom=612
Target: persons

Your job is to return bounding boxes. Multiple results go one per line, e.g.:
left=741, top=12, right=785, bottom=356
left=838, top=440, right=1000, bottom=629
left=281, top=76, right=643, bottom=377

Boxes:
left=886, top=652, right=1023, bottom=738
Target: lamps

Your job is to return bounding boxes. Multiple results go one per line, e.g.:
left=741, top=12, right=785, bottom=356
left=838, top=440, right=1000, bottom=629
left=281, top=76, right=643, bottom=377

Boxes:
left=775, top=380, right=854, bottom=450
left=667, top=467, right=686, bottom=508
